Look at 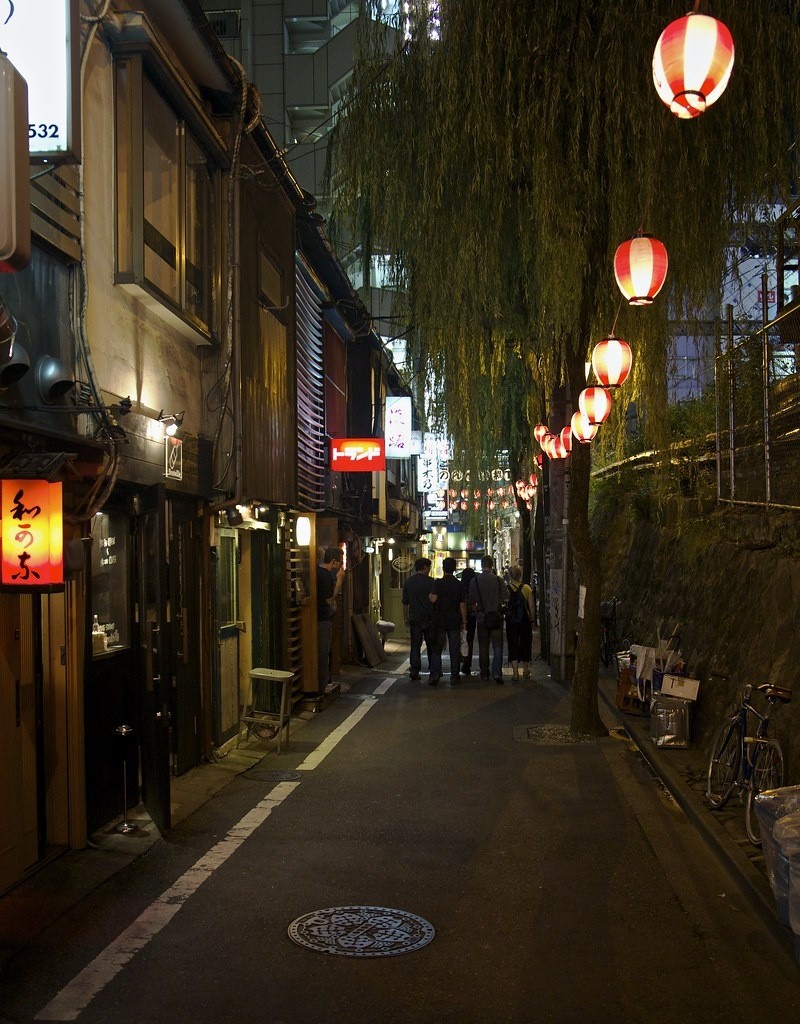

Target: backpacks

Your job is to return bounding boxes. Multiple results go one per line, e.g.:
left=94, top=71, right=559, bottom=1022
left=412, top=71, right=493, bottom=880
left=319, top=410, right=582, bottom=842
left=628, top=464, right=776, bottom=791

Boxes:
left=504, top=583, right=527, bottom=625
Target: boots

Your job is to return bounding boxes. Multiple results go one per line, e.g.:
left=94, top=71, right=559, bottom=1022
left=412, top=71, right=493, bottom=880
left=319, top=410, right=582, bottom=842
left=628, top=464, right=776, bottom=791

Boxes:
left=522, top=661, right=531, bottom=678
left=510, top=660, right=519, bottom=681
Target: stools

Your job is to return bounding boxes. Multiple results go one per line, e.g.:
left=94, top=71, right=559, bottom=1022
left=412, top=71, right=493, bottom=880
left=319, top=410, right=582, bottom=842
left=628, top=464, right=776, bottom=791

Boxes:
left=376, top=620, right=395, bottom=647
left=236, top=668, right=295, bottom=755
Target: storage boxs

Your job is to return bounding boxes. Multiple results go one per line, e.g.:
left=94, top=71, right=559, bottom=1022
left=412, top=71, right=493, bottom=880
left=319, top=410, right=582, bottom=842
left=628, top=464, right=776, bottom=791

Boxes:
left=653, top=668, right=690, bottom=691
left=661, top=674, right=700, bottom=701
left=617, top=668, right=650, bottom=717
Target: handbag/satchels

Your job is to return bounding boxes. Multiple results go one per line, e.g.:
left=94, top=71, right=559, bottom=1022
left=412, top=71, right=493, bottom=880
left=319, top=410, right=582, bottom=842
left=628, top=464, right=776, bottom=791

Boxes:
left=459, top=629, right=468, bottom=657
left=485, top=611, right=500, bottom=630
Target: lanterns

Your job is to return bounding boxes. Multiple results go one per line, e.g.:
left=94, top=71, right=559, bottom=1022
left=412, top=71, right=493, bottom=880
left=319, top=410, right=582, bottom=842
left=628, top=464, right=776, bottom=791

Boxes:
left=508, top=474, right=537, bottom=510
left=534, top=424, right=572, bottom=460
left=612, top=230, right=670, bottom=304
left=579, top=387, right=612, bottom=427
left=571, top=411, right=599, bottom=443
left=592, top=338, right=633, bottom=391
left=437, top=487, right=508, bottom=511
left=650, top=14, right=736, bottom=118
left=532, top=453, right=544, bottom=470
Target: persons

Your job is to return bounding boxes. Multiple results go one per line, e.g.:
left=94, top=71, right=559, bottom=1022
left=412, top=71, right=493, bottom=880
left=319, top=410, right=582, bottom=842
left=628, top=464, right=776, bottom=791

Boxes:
left=427, top=557, right=464, bottom=686
left=502, top=566, right=537, bottom=680
left=401, top=558, right=437, bottom=679
left=462, top=556, right=508, bottom=681
left=316, top=547, right=344, bottom=685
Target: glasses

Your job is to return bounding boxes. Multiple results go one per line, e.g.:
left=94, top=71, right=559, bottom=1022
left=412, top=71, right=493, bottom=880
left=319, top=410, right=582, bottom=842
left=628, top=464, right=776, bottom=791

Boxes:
left=332, top=558, right=343, bottom=565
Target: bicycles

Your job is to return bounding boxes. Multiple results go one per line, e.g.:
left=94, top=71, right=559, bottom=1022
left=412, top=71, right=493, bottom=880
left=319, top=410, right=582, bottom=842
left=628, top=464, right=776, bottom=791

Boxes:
left=601, top=595, right=631, bottom=671
left=703, top=671, right=792, bottom=847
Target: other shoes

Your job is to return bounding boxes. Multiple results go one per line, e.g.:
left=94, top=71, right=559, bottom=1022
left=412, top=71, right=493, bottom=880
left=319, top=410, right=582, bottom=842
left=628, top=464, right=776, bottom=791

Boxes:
left=450, top=675, right=460, bottom=685
left=410, top=668, right=421, bottom=680
left=461, top=664, right=471, bottom=675
left=492, top=674, right=504, bottom=684
left=480, top=670, right=490, bottom=680
left=428, top=672, right=441, bottom=685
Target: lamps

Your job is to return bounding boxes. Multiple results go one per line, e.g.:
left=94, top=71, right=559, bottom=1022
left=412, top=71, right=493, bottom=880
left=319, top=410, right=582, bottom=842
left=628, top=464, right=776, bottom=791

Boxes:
left=225, top=490, right=244, bottom=527
